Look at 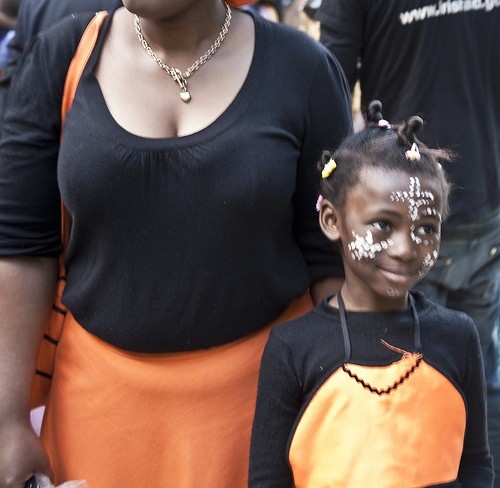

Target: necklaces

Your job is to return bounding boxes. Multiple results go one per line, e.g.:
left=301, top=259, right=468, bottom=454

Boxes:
left=336, top=289, right=424, bottom=396
left=134, top=2, right=231, bottom=104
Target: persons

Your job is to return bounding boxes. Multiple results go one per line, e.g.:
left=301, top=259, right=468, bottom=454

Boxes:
left=318, top=0, right=500, bottom=488
left=1, top=0, right=355, bottom=488
left=247, top=99, right=497, bottom=488
